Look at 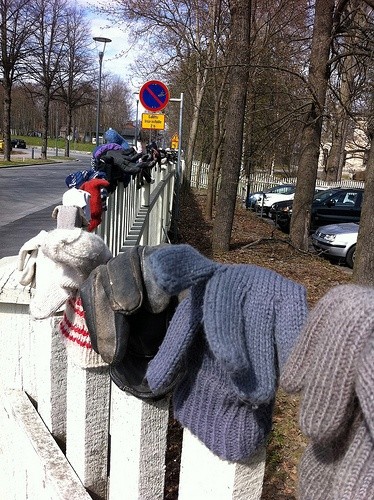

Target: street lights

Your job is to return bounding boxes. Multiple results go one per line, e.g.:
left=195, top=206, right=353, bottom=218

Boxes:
left=92, top=34, right=112, bottom=148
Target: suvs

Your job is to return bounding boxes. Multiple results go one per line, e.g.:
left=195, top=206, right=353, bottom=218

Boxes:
left=278, top=187, right=366, bottom=230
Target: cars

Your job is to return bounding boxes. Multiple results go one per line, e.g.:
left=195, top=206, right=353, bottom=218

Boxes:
left=260, top=192, right=296, bottom=216
left=250, top=184, right=292, bottom=203
left=314, top=220, right=360, bottom=266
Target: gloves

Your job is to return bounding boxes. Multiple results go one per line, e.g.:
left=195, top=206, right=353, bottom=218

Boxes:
left=277, top=284, right=374, bottom=500
left=99, top=147, right=150, bottom=174
left=146, top=243, right=308, bottom=462
left=79, top=245, right=186, bottom=400
left=17, top=228, right=110, bottom=320
